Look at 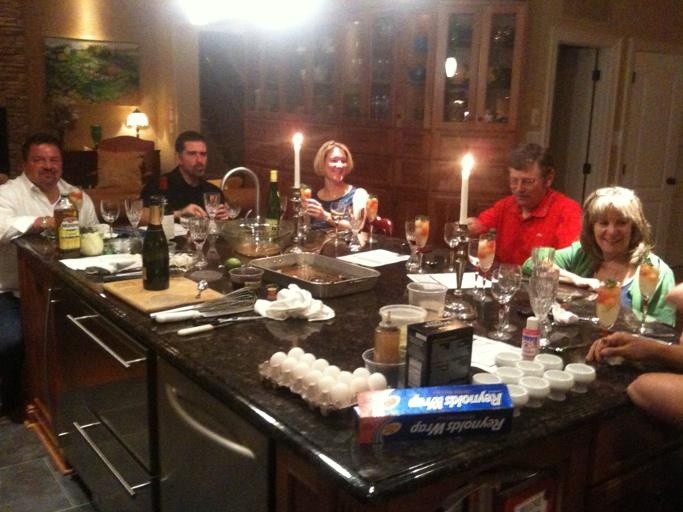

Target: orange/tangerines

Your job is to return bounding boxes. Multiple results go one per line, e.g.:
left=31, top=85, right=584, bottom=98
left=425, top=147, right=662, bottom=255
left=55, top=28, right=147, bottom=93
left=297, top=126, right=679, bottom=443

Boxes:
left=225, top=257, right=242, bottom=269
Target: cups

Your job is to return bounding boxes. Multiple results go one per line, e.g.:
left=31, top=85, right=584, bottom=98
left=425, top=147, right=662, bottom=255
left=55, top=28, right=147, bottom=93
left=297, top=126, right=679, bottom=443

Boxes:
left=80, top=232, right=103, bottom=255
left=229, top=266, right=264, bottom=291
left=471, top=350, right=596, bottom=418
left=361, top=281, right=446, bottom=388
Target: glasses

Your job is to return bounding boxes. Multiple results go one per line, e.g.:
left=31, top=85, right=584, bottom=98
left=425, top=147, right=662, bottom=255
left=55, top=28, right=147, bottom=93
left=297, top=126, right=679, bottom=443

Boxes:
left=510, top=177, right=543, bottom=186
left=593, top=186, right=634, bottom=198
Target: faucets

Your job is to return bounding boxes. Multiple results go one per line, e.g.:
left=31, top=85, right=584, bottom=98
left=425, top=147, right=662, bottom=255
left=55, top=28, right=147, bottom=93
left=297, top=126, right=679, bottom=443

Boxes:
left=220, top=166, right=262, bottom=224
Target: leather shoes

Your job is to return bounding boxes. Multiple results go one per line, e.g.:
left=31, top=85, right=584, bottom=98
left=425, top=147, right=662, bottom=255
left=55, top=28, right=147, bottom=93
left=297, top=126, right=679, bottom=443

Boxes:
left=2, top=402, right=25, bottom=423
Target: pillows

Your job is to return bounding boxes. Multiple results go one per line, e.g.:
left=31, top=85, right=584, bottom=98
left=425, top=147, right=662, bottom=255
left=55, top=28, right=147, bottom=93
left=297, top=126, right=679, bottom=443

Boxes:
left=95, top=148, right=146, bottom=187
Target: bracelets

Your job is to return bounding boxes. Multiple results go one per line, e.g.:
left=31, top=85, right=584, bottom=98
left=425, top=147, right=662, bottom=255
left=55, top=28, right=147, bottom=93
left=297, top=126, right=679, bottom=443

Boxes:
left=40, top=214, right=49, bottom=229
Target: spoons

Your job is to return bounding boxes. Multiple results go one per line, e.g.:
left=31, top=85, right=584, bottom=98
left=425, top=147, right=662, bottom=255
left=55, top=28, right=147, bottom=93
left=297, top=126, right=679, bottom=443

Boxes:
left=194, top=279, right=209, bottom=300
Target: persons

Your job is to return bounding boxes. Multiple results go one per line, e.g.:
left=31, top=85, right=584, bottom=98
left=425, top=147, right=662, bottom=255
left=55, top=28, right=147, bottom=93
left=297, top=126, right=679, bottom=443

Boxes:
left=300, top=138, right=370, bottom=233
left=139, top=128, right=233, bottom=221
left=585, top=328, right=683, bottom=428
left=519, top=183, right=677, bottom=329
left=465, top=140, right=584, bottom=275
left=0, top=134, right=100, bottom=420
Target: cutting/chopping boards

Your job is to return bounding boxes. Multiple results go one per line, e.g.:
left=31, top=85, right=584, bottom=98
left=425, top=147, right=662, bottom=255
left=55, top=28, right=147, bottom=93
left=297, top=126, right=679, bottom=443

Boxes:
left=102, top=276, right=223, bottom=312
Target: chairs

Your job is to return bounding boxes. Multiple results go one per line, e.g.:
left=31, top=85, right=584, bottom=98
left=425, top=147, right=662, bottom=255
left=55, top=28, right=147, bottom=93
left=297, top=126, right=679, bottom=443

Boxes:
left=79, top=136, right=154, bottom=216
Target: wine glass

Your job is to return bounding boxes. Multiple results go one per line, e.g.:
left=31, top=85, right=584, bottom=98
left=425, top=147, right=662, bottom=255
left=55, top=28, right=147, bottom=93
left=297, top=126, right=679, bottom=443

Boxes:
left=405, top=214, right=662, bottom=350
left=300, top=184, right=378, bottom=252
left=100, top=192, right=243, bottom=268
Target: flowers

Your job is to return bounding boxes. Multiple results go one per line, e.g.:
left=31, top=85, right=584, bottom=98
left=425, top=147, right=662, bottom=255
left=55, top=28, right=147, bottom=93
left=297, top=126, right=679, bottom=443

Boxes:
left=47, top=107, right=77, bottom=129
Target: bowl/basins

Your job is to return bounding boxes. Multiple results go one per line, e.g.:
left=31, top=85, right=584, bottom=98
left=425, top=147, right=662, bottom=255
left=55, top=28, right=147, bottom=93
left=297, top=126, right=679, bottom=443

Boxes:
left=225, top=218, right=294, bottom=258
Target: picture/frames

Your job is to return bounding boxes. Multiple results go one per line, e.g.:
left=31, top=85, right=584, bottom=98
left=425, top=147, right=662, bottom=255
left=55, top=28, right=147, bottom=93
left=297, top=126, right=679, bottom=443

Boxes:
left=42, top=34, right=143, bottom=106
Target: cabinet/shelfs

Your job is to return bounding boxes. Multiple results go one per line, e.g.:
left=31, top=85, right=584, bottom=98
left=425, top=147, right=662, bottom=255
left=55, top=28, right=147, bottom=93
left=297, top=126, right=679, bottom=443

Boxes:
left=596, top=409, right=683, bottom=512
left=388, top=416, right=596, bottom=512
left=59, top=150, right=162, bottom=192
left=18, top=249, right=57, bottom=456
left=276, top=437, right=369, bottom=512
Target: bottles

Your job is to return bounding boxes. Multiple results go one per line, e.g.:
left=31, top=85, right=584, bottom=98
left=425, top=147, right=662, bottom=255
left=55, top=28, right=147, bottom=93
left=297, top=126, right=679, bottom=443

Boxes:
left=264, top=171, right=281, bottom=237
left=54, top=193, right=80, bottom=252
left=141, top=200, right=171, bottom=290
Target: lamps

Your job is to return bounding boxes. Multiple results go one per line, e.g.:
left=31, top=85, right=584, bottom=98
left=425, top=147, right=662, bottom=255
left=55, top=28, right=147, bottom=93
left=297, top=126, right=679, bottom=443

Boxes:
left=126, top=108, right=149, bottom=138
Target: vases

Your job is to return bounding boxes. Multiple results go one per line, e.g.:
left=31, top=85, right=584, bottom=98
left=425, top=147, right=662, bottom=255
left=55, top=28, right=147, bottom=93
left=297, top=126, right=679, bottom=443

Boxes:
left=59, top=130, right=66, bottom=150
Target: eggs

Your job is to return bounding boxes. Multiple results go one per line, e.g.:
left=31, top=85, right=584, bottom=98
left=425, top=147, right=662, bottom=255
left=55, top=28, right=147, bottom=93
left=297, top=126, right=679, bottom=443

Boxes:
left=331, top=382, right=353, bottom=401
left=312, top=358, right=329, bottom=373
left=367, top=372, right=386, bottom=392
left=270, top=351, right=285, bottom=367
left=306, top=370, right=319, bottom=383
left=320, top=376, right=336, bottom=391
left=352, top=368, right=370, bottom=380
left=281, top=360, right=295, bottom=372
left=325, top=365, right=340, bottom=379
left=288, top=345, right=304, bottom=361
left=336, top=371, right=352, bottom=383
left=352, top=375, right=366, bottom=392
left=301, top=353, right=316, bottom=368
left=295, top=362, right=307, bottom=377
